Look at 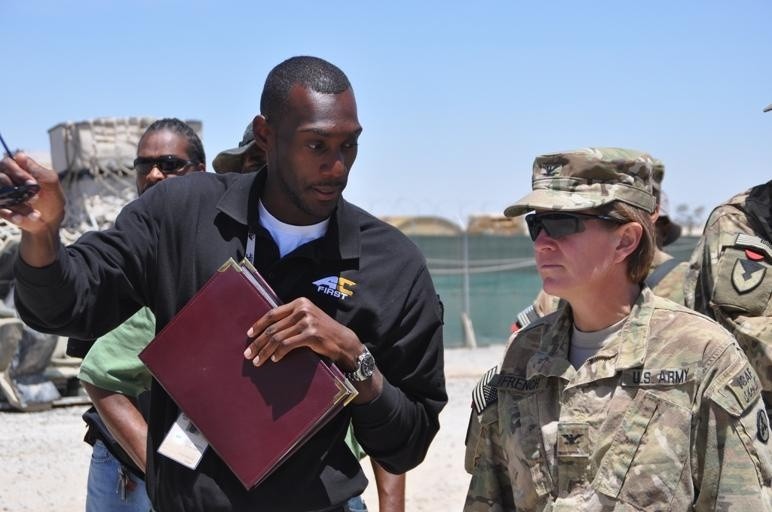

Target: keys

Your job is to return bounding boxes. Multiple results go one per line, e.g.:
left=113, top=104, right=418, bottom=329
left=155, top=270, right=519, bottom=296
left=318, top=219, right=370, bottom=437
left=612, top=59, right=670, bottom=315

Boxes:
left=113, top=465, right=137, bottom=501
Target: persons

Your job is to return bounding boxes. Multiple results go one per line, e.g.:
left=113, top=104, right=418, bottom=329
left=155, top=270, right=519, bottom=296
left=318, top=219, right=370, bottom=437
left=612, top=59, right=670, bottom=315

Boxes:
left=66, top=117, right=208, bottom=512
left=457, top=146, right=771, bottom=512
left=0, top=55, right=448, bottom=512
left=77, top=112, right=406, bottom=512
left=648, top=177, right=771, bottom=429
left=509, top=209, right=680, bottom=339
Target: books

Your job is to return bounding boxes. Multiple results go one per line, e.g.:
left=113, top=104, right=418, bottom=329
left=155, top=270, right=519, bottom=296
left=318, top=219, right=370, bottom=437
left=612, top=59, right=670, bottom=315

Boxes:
left=137, top=258, right=360, bottom=494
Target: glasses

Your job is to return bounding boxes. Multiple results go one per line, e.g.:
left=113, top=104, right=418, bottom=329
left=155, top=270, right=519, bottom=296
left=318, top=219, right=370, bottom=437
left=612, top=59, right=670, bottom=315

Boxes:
left=134, top=155, right=197, bottom=174
left=525, top=213, right=617, bottom=240
left=1, top=131, right=40, bottom=205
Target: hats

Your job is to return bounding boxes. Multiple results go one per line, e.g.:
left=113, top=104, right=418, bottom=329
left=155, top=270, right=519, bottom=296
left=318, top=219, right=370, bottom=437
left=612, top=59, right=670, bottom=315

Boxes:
left=504, top=147, right=665, bottom=218
left=763, top=104, right=771, bottom=111
left=212, top=120, right=256, bottom=173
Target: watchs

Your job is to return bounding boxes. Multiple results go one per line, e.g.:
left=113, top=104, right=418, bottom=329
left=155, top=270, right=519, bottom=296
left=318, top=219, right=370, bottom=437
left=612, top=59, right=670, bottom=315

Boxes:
left=342, top=342, right=376, bottom=383
